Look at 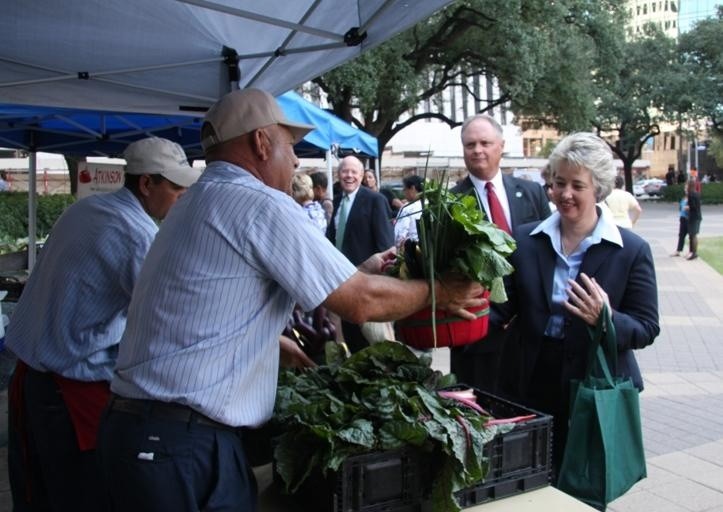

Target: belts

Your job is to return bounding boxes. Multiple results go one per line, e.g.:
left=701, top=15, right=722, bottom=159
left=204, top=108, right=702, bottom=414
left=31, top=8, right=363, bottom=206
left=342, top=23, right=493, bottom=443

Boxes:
left=114, top=396, right=218, bottom=426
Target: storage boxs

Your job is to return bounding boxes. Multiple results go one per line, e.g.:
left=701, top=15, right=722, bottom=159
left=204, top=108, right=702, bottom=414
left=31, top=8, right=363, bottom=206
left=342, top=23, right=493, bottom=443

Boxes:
left=333, top=384, right=553, bottom=512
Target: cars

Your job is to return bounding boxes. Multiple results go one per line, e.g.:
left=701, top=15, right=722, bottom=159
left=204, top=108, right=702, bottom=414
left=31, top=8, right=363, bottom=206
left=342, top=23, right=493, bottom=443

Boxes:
left=379, top=179, right=403, bottom=191
left=640, top=179, right=668, bottom=197
left=632, top=176, right=662, bottom=197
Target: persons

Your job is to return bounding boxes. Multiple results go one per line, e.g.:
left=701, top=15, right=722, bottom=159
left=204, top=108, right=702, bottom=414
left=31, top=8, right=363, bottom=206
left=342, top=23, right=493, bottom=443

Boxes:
left=479, top=132, right=660, bottom=473
left=665, top=166, right=717, bottom=261
left=4, top=135, right=201, bottom=512
left=310, top=172, right=334, bottom=228
left=361, top=169, right=404, bottom=214
left=289, top=173, right=328, bottom=236
left=604, top=175, right=642, bottom=231
left=97, top=86, right=489, bottom=512
left=546, top=184, right=558, bottom=214
left=445, top=114, right=553, bottom=405
left=324, top=155, right=395, bottom=352
left=394, top=174, right=430, bottom=255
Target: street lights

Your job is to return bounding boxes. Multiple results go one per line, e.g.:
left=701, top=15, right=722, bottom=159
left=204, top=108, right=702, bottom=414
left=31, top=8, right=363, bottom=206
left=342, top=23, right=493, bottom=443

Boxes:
left=693, top=145, right=705, bottom=170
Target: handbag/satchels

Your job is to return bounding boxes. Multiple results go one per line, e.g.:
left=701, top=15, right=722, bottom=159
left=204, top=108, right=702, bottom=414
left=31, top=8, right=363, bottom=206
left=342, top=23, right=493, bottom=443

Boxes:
left=552, top=301, right=646, bottom=512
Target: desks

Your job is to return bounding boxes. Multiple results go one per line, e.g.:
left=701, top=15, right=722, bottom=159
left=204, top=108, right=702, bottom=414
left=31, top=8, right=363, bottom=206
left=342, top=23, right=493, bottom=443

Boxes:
left=461, top=488, right=603, bottom=512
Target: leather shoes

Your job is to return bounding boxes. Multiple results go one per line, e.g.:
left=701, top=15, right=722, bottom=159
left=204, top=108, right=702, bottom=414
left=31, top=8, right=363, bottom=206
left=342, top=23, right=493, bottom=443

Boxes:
left=671, top=251, right=697, bottom=260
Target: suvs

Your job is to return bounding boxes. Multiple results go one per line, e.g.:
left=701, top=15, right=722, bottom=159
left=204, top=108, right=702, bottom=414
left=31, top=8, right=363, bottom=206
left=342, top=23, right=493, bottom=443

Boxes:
left=507, top=165, right=550, bottom=202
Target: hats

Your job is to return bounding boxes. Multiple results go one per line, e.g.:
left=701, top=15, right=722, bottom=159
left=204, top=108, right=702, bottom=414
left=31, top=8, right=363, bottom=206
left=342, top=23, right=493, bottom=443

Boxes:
left=201, top=87, right=317, bottom=147
left=123, top=136, right=203, bottom=188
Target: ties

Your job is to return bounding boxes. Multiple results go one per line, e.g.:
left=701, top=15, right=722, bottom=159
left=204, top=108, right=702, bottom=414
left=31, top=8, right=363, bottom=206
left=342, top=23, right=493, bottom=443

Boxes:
left=336, top=196, right=348, bottom=252
left=485, top=183, right=511, bottom=236
